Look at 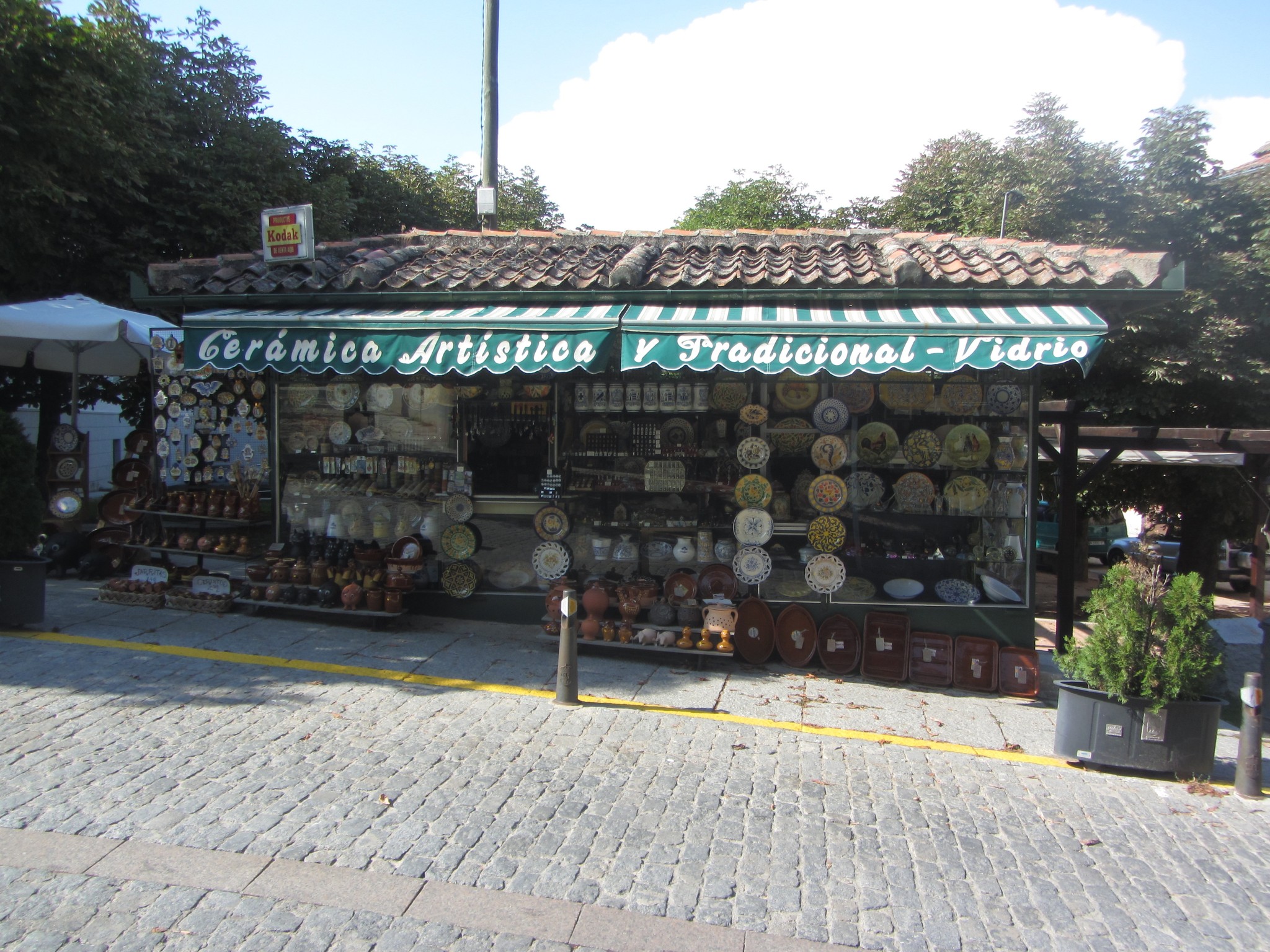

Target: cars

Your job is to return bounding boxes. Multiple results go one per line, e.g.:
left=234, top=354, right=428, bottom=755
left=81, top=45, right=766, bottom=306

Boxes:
left=1036, top=500, right=1129, bottom=567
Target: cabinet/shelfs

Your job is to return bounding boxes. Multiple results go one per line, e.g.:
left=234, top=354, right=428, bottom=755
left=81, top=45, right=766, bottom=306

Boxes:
left=123, top=506, right=271, bottom=562
left=233, top=580, right=415, bottom=629
left=833, top=412, right=1028, bottom=563
left=536, top=612, right=735, bottom=671
left=42, top=430, right=89, bottom=582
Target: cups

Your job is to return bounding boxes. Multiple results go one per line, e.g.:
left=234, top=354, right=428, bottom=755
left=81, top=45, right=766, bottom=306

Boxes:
left=1005, top=532, right=1022, bottom=560
left=698, top=530, right=714, bottom=563
left=366, top=586, right=402, bottom=612
left=593, top=537, right=610, bottom=560
left=320, top=443, right=330, bottom=453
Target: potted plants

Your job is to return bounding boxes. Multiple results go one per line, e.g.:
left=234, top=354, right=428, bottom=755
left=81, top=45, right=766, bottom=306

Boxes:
left=0, top=409, right=53, bottom=633
left=1052, top=562, right=1229, bottom=777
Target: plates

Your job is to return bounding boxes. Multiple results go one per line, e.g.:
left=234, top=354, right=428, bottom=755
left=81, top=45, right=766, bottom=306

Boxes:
left=714, top=370, right=771, bottom=584
left=441, top=494, right=477, bottom=597
left=532, top=507, right=571, bottom=580
left=580, top=418, right=694, bottom=450
left=51, top=423, right=150, bottom=551
left=665, top=565, right=1041, bottom=696
left=286, top=375, right=509, bottom=453
left=531, top=365, right=556, bottom=381
left=772, top=369, right=1022, bottom=604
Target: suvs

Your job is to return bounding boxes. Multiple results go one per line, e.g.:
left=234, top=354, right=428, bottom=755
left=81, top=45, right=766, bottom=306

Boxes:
left=1105, top=513, right=1270, bottom=594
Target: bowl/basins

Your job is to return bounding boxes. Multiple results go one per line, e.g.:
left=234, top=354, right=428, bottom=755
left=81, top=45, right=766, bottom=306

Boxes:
left=980, top=574, right=1021, bottom=604
left=641, top=540, right=672, bottom=560
left=354, top=536, right=425, bottom=589
left=455, top=383, right=553, bottom=399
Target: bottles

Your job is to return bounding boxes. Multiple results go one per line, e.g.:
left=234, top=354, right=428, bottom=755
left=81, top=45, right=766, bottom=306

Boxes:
left=611, top=533, right=747, bottom=562
left=178, top=492, right=417, bottom=594
left=797, top=542, right=821, bottom=564
left=995, top=435, right=1029, bottom=470
left=575, top=383, right=711, bottom=413
left=998, top=480, right=1028, bottom=516
left=543, top=568, right=739, bottom=653
